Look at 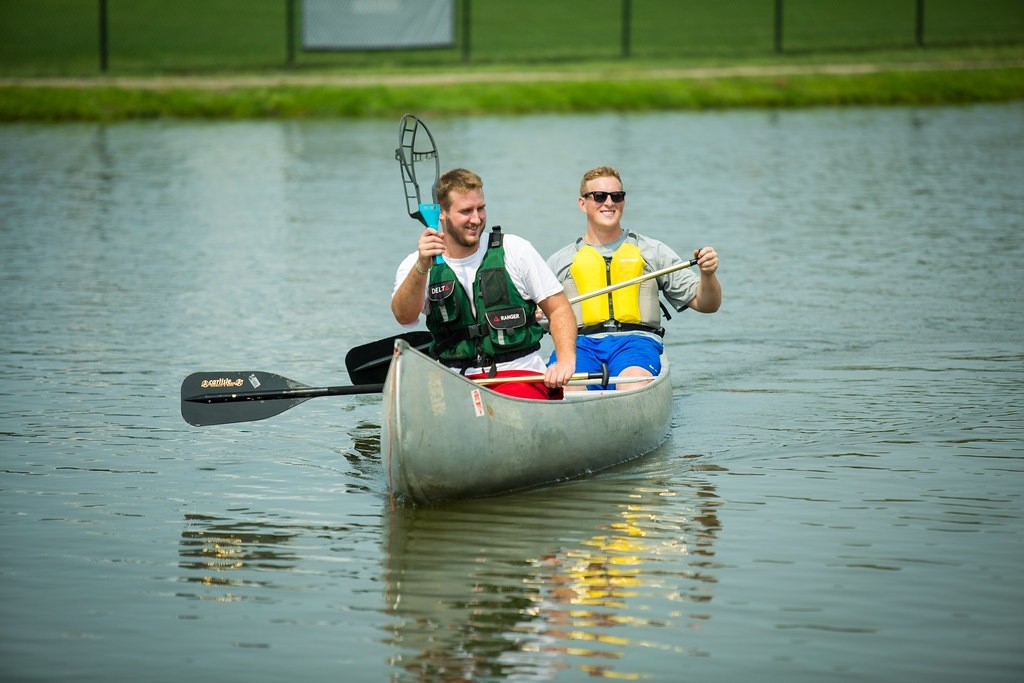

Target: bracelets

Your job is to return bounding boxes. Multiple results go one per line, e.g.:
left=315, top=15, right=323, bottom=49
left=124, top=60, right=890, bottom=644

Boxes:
left=415, top=263, right=428, bottom=274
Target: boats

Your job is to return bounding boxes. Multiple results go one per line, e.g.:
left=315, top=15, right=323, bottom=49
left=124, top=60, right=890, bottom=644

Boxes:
left=382, top=339, right=673, bottom=509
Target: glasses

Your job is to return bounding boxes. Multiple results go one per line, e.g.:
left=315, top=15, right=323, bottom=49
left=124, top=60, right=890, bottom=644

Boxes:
left=581, top=191, right=627, bottom=203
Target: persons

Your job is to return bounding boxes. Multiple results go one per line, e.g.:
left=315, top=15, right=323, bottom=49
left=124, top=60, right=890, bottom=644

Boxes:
left=534, top=165, right=721, bottom=392
left=392, top=170, right=577, bottom=401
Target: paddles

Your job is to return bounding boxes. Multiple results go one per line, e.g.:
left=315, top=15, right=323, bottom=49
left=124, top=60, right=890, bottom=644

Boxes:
left=180, top=361, right=608, bottom=428
left=345, top=248, right=703, bottom=386
